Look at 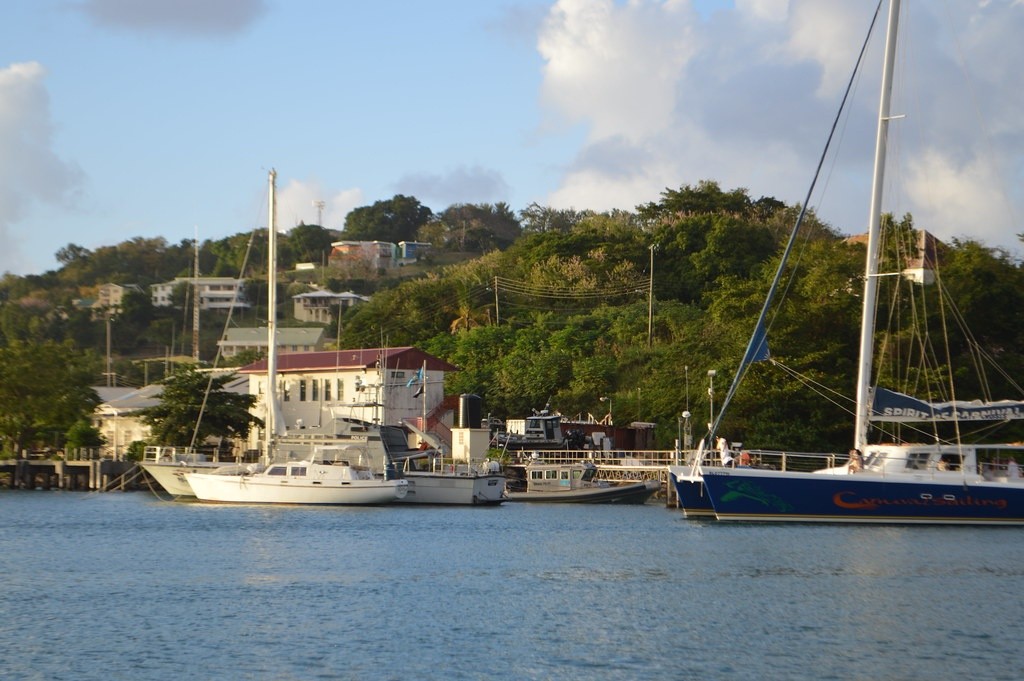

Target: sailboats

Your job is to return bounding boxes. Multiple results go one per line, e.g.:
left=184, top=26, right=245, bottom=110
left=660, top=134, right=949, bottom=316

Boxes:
left=666, top=1, right=1024, bottom=527
left=138, top=164, right=510, bottom=508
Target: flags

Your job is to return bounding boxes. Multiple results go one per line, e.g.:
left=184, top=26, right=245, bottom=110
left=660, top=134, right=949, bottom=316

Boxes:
left=413, top=386, right=424, bottom=398
left=406, top=365, right=424, bottom=388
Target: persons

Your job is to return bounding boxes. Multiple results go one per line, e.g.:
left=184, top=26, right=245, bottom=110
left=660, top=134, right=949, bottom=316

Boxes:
left=848, top=448, right=866, bottom=472
left=1006, top=456, right=1019, bottom=480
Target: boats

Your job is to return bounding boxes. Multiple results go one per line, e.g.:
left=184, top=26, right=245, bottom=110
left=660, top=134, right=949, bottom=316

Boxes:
left=868, top=386, right=1024, bottom=447
left=181, top=447, right=409, bottom=506
left=495, top=394, right=591, bottom=459
left=506, top=462, right=661, bottom=506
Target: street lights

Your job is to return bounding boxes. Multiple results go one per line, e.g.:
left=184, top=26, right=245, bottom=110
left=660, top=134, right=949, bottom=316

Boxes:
left=600, top=398, right=612, bottom=426
left=706, top=368, right=716, bottom=467
